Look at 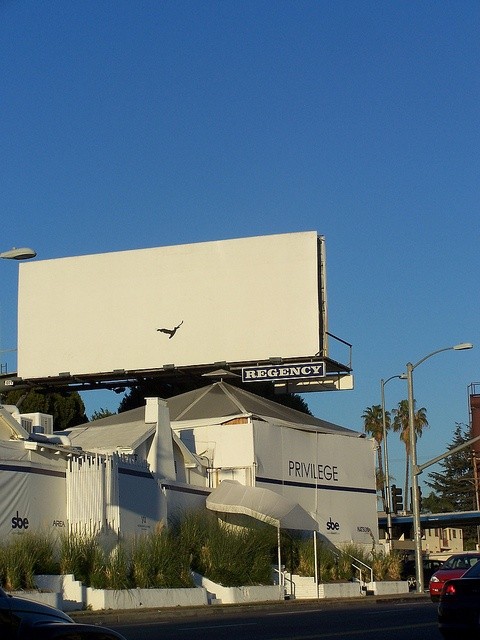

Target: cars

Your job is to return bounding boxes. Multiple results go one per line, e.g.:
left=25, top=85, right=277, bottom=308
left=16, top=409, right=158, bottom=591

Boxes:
left=0, top=586, right=75, bottom=622
left=0, top=606, right=127, bottom=640
left=435, top=558, right=480, bottom=640
left=429, top=554, right=480, bottom=602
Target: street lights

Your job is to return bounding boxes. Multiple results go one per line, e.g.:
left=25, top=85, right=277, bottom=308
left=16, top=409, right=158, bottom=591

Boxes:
left=0, top=248, right=36, bottom=259
left=380, top=374, right=409, bottom=540
left=407, top=343, right=473, bottom=595
